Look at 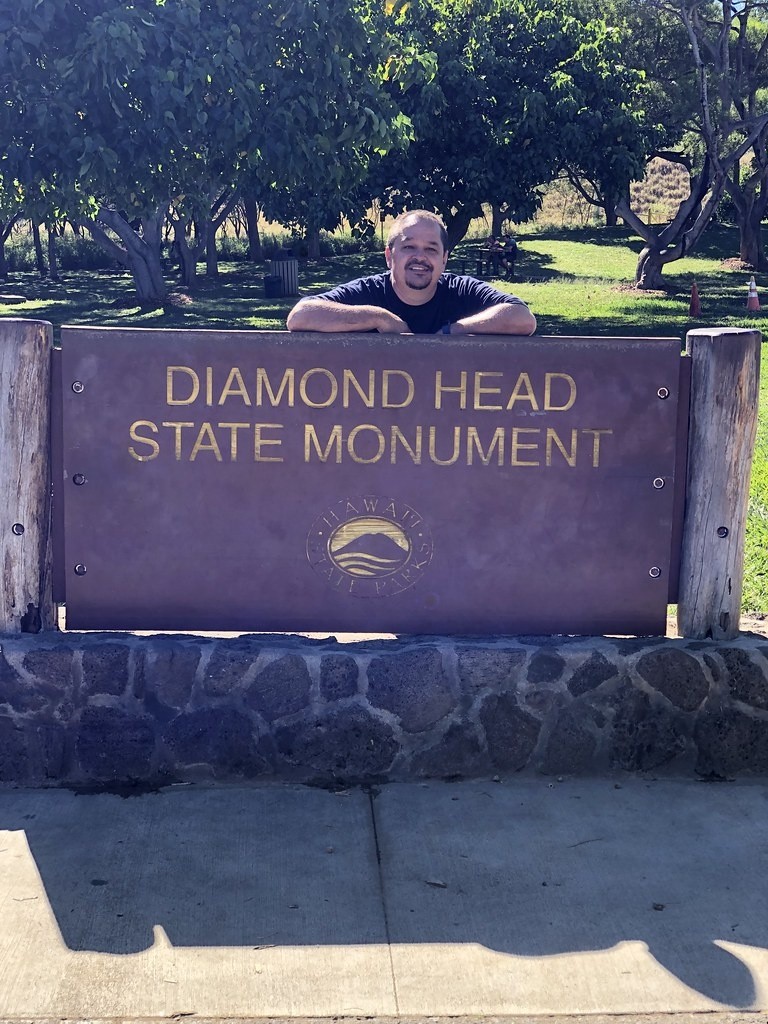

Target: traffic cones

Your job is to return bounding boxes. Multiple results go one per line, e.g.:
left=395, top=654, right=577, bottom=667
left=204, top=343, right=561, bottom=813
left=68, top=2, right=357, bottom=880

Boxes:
left=747, top=276, right=760, bottom=311
left=688, top=283, right=701, bottom=316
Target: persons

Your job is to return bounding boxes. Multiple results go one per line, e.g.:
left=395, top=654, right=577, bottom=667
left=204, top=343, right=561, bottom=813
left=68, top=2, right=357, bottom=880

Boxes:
left=287, top=208, right=538, bottom=337
left=484, top=234, right=517, bottom=276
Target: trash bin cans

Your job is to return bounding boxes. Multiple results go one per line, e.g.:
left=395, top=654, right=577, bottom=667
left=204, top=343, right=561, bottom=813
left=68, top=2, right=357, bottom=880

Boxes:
left=270, top=247, right=299, bottom=297
left=263, top=275, right=281, bottom=298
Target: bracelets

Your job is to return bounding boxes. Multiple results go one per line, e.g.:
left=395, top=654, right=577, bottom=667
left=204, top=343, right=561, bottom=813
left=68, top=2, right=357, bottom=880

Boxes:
left=440, top=320, right=453, bottom=334
